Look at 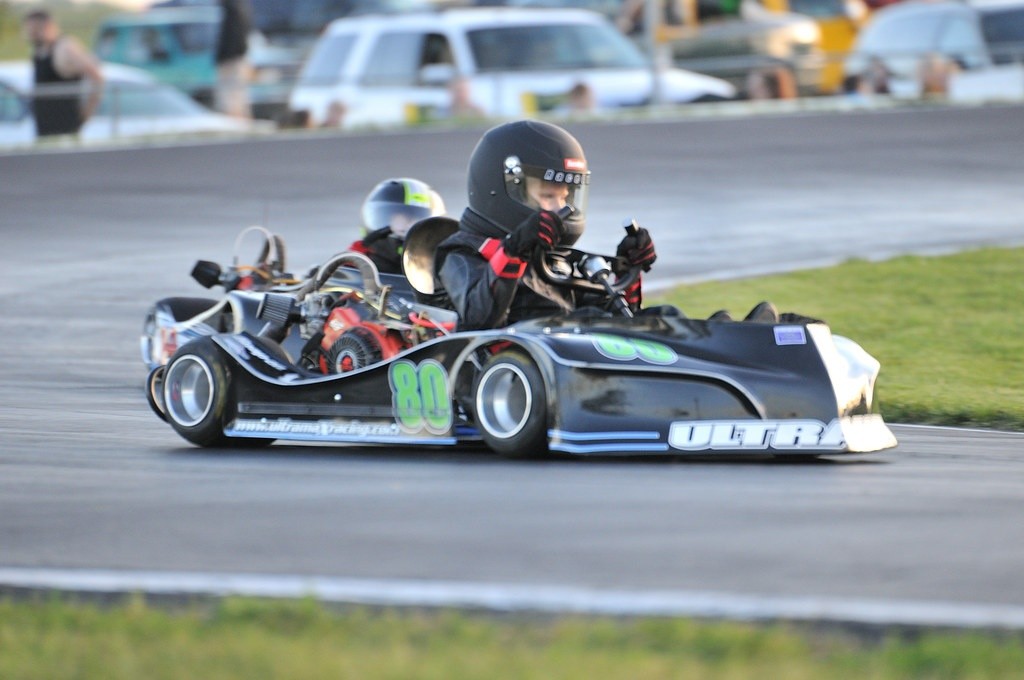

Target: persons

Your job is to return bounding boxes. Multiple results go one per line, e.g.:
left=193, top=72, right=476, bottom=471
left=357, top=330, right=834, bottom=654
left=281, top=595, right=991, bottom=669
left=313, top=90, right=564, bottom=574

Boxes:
left=22, top=12, right=104, bottom=137
left=432, top=120, right=779, bottom=333
left=212, top=0, right=256, bottom=119
left=348, top=176, right=448, bottom=274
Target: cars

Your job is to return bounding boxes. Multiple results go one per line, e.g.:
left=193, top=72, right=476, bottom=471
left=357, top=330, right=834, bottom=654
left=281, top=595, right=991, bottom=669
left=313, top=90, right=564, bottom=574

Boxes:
left=613, top=2, right=1024, bottom=101
left=89, top=10, right=277, bottom=96
left=0, top=59, right=277, bottom=142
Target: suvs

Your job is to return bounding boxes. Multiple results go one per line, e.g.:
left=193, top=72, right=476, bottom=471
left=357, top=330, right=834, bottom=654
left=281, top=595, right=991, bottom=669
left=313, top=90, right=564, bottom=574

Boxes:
left=279, top=2, right=742, bottom=129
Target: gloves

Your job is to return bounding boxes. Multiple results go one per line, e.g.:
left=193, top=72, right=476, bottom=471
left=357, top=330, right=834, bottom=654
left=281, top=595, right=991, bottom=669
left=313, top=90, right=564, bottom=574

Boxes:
left=615, top=226, right=656, bottom=280
left=503, top=208, right=564, bottom=262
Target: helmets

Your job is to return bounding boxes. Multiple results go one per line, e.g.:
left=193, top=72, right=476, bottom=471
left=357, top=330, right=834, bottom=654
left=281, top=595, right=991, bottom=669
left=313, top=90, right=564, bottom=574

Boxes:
left=466, top=119, right=589, bottom=250
left=361, top=178, right=445, bottom=265
